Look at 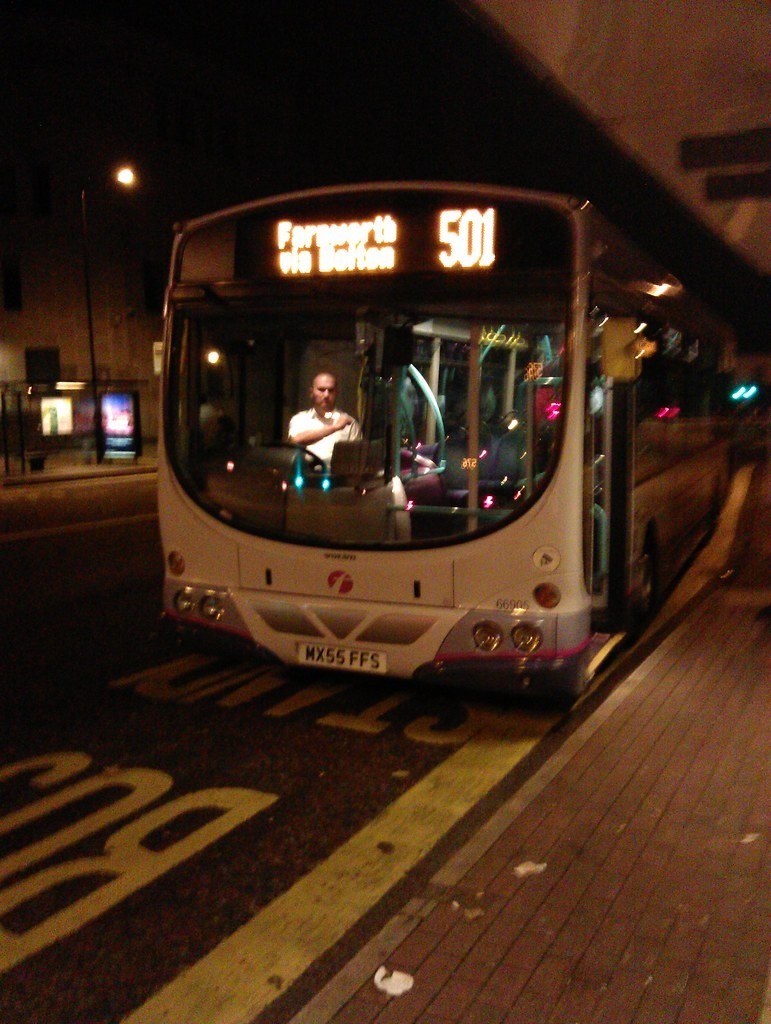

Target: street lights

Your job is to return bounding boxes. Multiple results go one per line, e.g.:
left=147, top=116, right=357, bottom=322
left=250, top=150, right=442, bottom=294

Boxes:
left=79, top=166, right=134, bottom=462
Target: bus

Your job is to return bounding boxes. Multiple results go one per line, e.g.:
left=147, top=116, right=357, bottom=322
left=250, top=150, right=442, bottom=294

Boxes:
left=151, top=176, right=742, bottom=715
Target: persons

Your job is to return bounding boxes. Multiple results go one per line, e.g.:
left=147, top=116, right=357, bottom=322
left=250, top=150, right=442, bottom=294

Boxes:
left=288, top=372, right=362, bottom=471
